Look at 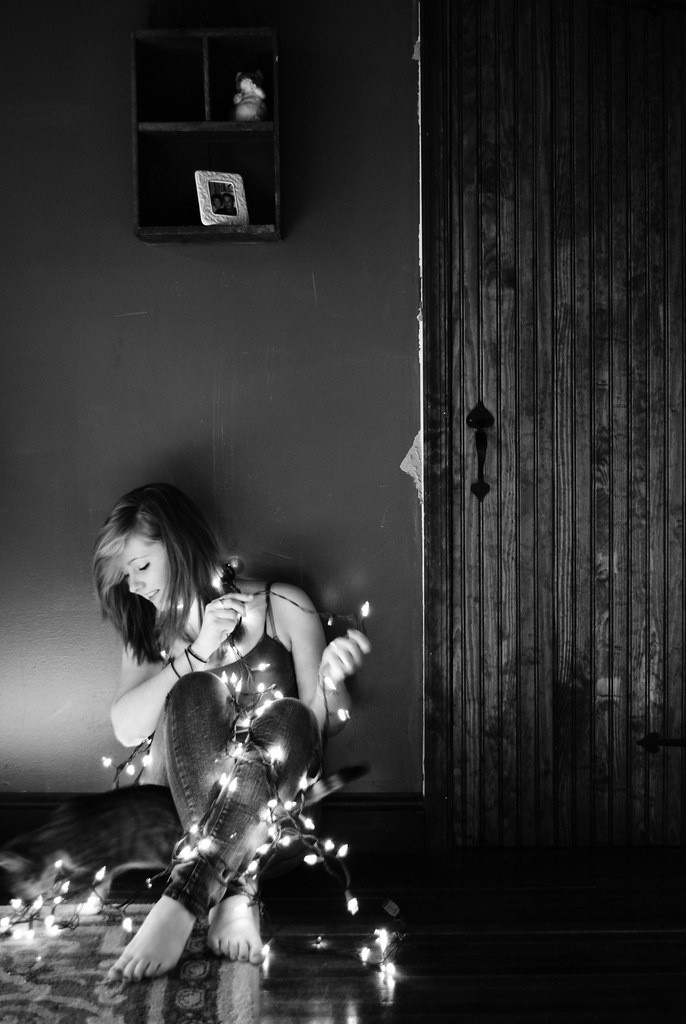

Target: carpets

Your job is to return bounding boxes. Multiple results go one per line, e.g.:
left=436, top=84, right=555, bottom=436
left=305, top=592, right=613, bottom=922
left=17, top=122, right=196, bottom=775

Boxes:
left=0, top=902, right=263, bottom=1024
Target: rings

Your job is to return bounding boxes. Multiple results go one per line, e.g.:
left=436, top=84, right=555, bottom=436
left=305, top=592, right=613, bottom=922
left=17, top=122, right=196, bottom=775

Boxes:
left=219, top=598, right=226, bottom=610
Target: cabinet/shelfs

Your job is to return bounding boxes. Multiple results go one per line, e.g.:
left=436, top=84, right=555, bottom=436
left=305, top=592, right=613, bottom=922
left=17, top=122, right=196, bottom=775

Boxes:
left=131, top=22, right=284, bottom=247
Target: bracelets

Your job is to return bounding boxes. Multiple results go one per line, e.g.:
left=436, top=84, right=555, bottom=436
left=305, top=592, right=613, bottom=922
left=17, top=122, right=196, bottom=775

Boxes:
left=317, top=675, right=344, bottom=697
left=168, top=658, right=180, bottom=679
left=188, top=645, right=207, bottom=663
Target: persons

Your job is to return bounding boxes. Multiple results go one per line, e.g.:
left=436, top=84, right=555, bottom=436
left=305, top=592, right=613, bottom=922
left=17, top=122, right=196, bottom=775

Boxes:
left=93, top=483, right=372, bottom=980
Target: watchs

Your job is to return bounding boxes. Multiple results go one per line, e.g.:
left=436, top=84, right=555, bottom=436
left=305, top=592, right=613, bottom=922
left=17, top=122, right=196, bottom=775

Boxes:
left=185, top=649, right=194, bottom=673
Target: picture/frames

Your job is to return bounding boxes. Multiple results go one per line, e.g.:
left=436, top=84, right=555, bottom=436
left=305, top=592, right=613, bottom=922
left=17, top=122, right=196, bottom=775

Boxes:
left=194, top=169, right=250, bottom=226
